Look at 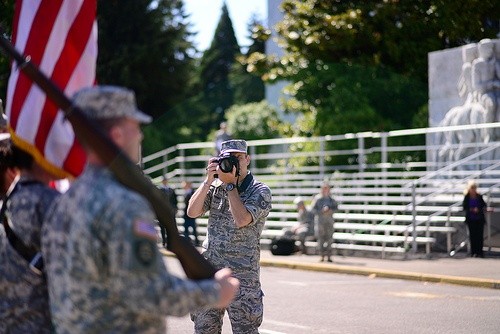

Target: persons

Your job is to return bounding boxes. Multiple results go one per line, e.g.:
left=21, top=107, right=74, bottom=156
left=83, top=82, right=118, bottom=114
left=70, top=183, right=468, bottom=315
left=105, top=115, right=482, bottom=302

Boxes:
left=0, top=131, right=64, bottom=334
left=159, top=174, right=202, bottom=248
left=434, top=38, right=500, bottom=178
left=269, top=181, right=339, bottom=263
left=41, top=86, right=241, bottom=334
left=187, top=139, right=273, bottom=334
left=462, top=181, right=487, bottom=258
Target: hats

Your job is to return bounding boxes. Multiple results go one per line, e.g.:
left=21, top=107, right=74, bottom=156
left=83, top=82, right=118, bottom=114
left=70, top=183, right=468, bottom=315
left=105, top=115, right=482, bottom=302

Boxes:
left=219, top=139, right=248, bottom=156
left=320, top=180, right=334, bottom=188
left=70, top=86, right=152, bottom=124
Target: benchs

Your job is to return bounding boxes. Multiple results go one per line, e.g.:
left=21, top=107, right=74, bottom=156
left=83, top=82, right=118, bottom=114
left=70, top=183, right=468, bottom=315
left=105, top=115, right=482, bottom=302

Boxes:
left=151, top=162, right=500, bottom=261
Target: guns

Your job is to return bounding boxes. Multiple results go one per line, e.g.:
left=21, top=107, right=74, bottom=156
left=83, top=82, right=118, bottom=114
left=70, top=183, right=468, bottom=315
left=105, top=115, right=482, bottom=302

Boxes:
left=0, top=32, right=225, bottom=282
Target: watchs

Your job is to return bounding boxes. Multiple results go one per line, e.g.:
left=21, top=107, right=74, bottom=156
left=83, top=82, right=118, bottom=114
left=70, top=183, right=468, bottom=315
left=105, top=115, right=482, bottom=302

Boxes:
left=224, top=183, right=238, bottom=195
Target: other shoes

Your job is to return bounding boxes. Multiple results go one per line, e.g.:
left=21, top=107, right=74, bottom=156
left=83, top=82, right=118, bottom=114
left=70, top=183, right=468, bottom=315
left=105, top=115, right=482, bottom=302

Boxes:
left=319, top=254, right=333, bottom=262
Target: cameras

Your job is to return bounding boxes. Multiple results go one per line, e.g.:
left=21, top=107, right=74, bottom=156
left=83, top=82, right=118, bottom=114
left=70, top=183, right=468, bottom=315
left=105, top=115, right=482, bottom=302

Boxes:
left=213, top=154, right=240, bottom=178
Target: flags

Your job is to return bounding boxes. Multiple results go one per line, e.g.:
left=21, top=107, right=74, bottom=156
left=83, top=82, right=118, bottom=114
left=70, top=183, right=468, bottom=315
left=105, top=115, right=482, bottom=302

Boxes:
left=6, top=0, right=98, bottom=181
